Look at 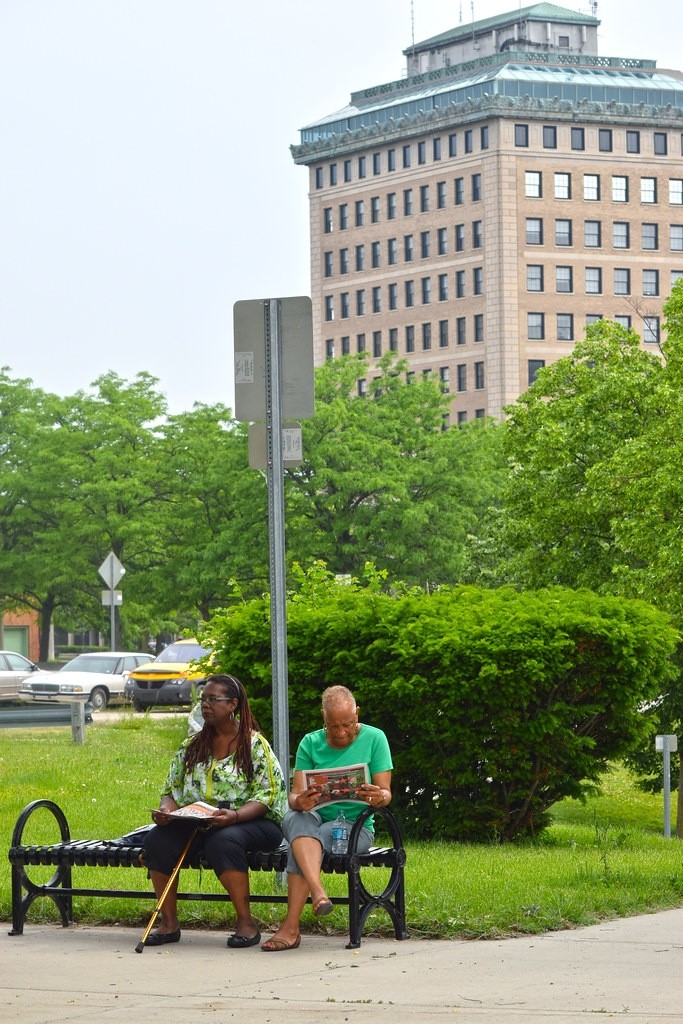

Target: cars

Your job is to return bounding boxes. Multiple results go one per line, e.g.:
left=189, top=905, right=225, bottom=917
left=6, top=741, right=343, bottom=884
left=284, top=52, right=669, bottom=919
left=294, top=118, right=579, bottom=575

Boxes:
left=0, top=650, right=45, bottom=703
left=17, top=652, right=156, bottom=713
left=124, top=638, right=223, bottom=714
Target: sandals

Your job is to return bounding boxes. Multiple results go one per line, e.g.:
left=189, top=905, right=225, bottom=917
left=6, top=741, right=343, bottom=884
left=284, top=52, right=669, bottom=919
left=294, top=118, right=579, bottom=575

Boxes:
left=261, top=933, right=302, bottom=951
left=312, top=897, right=335, bottom=916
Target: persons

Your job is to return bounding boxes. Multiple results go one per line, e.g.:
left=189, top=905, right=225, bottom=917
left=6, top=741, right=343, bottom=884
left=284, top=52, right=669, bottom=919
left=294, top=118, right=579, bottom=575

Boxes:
left=141, top=673, right=289, bottom=948
left=261, top=685, right=394, bottom=950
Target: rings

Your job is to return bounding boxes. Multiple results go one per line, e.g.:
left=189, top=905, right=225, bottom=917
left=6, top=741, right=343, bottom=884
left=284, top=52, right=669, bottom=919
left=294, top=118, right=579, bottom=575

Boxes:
left=369, top=797, right=371, bottom=802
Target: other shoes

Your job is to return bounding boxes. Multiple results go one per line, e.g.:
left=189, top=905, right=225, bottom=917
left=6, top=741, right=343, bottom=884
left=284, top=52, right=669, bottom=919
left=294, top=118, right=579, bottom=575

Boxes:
left=145, top=928, right=181, bottom=945
left=227, top=927, right=261, bottom=949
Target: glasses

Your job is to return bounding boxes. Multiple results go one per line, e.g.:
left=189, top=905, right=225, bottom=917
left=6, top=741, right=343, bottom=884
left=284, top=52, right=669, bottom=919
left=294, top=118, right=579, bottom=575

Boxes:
left=198, top=695, right=233, bottom=704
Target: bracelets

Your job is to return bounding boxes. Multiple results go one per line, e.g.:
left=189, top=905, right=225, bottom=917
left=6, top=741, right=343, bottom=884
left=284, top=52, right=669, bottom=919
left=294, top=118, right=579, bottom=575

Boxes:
left=377, top=791, right=386, bottom=805
left=235, top=810, right=239, bottom=824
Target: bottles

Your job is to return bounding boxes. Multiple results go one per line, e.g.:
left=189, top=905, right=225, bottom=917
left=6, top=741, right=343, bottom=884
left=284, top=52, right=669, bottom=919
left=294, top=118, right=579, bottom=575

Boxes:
left=332, top=810, right=349, bottom=855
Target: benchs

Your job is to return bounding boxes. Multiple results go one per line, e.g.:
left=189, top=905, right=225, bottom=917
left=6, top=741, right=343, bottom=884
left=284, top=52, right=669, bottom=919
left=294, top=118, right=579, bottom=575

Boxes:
left=8, top=799, right=410, bottom=949
